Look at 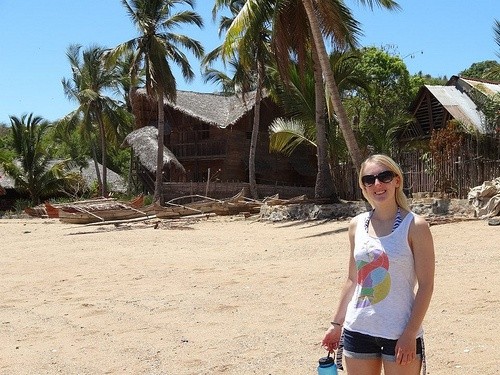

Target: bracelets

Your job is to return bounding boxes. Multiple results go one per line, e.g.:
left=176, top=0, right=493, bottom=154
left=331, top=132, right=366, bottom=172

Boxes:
left=330, top=322, right=342, bottom=328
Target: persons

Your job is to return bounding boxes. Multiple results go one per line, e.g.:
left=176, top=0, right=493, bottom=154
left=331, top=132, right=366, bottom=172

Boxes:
left=321, top=154, right=435, bottom=375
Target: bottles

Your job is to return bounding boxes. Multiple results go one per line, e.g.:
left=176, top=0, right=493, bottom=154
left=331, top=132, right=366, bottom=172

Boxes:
left=317, top=349, right=338, bottom=375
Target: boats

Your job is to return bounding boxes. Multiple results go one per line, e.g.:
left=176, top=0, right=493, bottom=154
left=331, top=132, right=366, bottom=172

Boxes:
left=210, top=195, right=307, bottom=215
left=44, top=192, right=144, bottom=218
left=153, top=187, right=245, bottom=219
left=58, top=197, right=161, bottom=224
left=24, top=191, right=115, bottom=217
left=243, top=193, right=279, bottom=204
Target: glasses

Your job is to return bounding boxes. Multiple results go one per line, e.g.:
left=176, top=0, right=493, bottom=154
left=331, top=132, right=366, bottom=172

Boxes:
left=361, top=170, right=398, bottom=187
